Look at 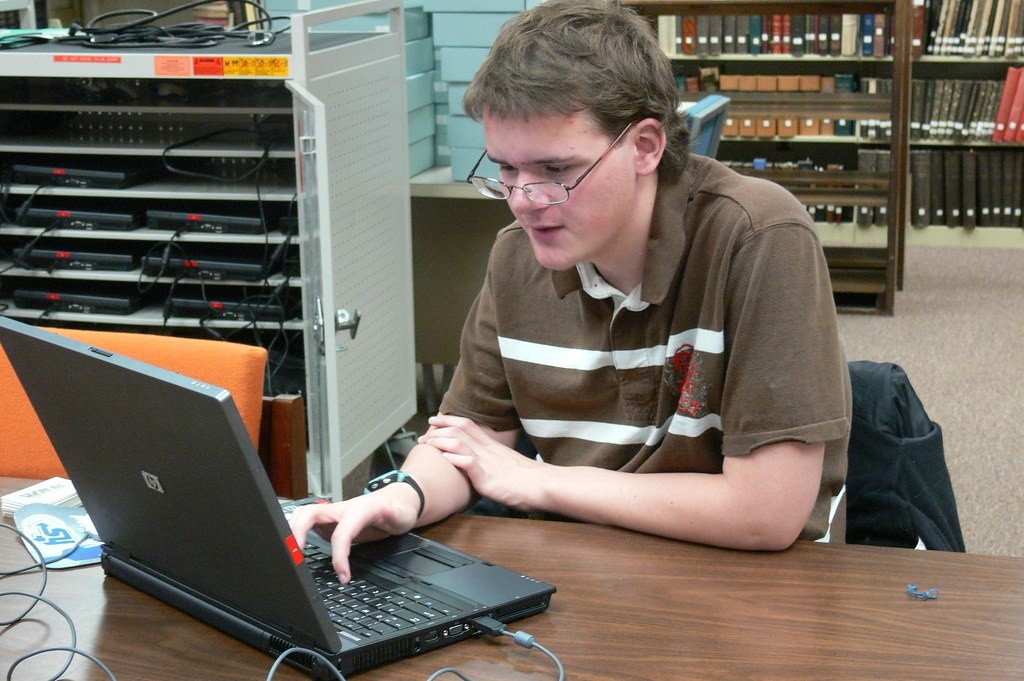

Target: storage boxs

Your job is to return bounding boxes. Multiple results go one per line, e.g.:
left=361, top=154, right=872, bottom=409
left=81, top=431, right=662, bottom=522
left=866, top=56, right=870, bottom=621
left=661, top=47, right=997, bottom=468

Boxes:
left=264, top=0, right=545, bottom=183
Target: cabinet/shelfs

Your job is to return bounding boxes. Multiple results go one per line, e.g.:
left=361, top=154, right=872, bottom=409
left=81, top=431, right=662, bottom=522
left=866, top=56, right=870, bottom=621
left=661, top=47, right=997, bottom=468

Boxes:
left=622, top=0, right=913, bottom=316
left=656, top=15, right=1024, bottom=248
left=0, top=0, right=419, bottom=498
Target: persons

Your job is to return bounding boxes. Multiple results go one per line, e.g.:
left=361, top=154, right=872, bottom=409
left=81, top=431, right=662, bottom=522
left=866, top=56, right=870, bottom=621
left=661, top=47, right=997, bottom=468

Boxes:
left=283, top=0, right=852, bottom=586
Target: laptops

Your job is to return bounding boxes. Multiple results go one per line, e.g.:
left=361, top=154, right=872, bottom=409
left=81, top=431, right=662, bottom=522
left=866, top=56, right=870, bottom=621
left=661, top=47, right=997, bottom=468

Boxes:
left=12, top=152, right=296, bottom=322
left=0, top=316, right=557, bottom=681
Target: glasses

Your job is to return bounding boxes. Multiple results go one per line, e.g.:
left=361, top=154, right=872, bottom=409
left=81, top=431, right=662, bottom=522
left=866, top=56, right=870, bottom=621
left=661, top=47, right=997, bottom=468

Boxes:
left=467, top=121, right=640, bottom=208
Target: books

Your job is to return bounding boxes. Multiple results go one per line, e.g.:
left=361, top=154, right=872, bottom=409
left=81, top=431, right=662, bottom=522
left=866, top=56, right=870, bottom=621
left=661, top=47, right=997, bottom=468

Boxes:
left=676, top=68, right=891, bottom=222
left=910, top=67, right=1024, bottom=143
left=910, top=150, right=1024, bottom=230
left=657, top=14, right=895, bottom=57
left=911, top=0, right=1024, bottom=58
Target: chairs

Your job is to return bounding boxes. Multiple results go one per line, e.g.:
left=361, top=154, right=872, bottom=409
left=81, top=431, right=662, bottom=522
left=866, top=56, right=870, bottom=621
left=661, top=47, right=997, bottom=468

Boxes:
left=261, top=394, right=310, bottom=502
left=686, top=94, right=732, bottom=159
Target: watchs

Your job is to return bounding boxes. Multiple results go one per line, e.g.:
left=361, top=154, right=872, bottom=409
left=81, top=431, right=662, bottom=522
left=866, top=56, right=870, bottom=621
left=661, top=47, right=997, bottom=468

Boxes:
left=363, top=470, right=425, bottom=519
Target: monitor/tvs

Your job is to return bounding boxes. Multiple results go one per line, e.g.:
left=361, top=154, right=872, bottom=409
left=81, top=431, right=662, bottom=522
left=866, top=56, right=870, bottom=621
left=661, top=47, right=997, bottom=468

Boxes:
left=676, top=94, right=730, bottom=160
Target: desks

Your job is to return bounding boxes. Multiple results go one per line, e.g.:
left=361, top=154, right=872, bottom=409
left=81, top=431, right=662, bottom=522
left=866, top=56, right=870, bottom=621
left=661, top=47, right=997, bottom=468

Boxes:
left=0, top=476, right=1024, bottom=681
left=408, top=165, right=515, bottom=366
